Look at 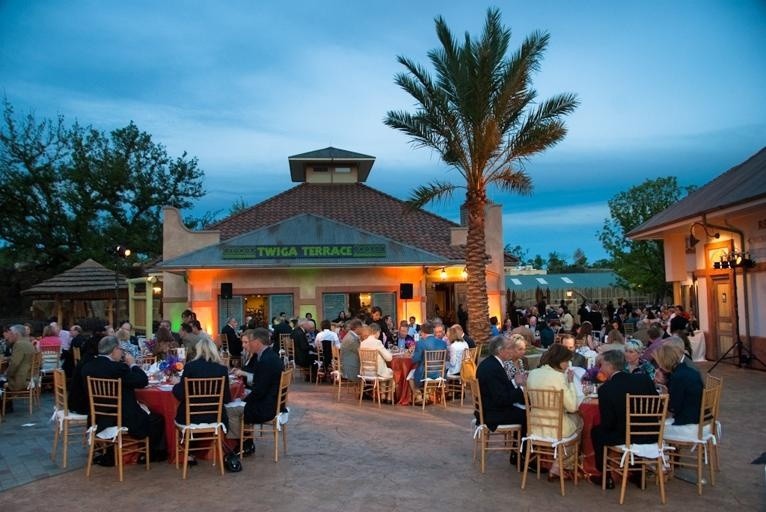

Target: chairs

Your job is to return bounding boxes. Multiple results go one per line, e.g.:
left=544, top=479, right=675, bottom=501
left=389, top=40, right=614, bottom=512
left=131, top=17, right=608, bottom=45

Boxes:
left=602, top=390, right=670, bottom=504
left=50, top=368, right=89, bottom=467
left=658, top=374, right=724, bottom=494
left=84, top=374, right=150, bottom=482
left=274, top=320, right=484, bottom=410
left=523, top=318, right=706, bottom=367
left=521, top=385, right=582, bottom=496
left=468, top=377, right=523, bottom=475
left=173, top=374, right=227, bottom=479
left=238, top=365, right=295, bottom=463
left=3, top=320, right=235, bottom=417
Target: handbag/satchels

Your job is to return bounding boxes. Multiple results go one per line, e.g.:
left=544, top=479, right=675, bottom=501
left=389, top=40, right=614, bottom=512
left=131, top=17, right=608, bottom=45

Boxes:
left=223, top=451, right=242, bottom=472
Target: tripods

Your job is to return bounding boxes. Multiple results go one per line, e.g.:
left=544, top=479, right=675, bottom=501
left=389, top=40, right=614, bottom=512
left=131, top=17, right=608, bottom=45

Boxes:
left=706, top=269, right=766, bottom=374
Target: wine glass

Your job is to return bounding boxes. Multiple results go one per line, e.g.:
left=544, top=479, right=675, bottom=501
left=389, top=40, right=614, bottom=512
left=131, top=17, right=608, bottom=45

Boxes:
left=582, top=381, right=595, bottom=401
left=118, top=349, right=188, bottom=388
left=388, top=341, right=414, bottom=357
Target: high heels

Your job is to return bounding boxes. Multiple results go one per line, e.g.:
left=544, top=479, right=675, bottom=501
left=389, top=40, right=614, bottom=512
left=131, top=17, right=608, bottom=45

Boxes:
left=188, top=455, right=198, bottom=468
left=549, top=470, right=570, bottom=482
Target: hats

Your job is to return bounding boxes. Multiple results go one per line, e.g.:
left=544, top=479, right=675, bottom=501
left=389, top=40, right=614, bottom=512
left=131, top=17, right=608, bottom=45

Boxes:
left=158, top=327, right=174, bottom=343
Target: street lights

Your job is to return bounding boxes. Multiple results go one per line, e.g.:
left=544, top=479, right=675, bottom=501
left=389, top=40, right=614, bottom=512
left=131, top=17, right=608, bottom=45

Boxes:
left=105, top=242, right=132, bottom=330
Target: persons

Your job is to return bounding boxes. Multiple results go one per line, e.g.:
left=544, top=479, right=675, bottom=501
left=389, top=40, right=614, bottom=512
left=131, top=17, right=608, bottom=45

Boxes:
left=1, top=317, right=91, bottom=413
left=474, top=294, right=707, bottom=488
left=62, top=310, right=285, bottom=466
left=271, top=304, right=475, bottom=407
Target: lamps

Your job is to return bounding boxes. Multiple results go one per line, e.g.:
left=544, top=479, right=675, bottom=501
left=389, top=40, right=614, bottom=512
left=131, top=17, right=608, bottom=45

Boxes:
left=105, top=244, right=131, bottom=258
left=689, top=222, right=719, bottom=247
left=706, top=249, right=765, bottom=373
left=426, top=266, right=448, bottom=279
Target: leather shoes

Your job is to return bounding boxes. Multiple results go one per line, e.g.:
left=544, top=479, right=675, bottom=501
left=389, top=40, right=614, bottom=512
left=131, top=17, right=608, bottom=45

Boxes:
left=591, top=476, right=615, bottom=489
left=234, top=444, right=255, bottom=453
left=510, top=453, right=517, bottom=465
left=630, top=476, right=646, bottom=488
left=521, top=463, right=547, bottom=473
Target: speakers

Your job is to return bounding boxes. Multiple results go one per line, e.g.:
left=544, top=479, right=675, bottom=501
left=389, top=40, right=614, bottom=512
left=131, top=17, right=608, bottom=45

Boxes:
left=221, top=283, right=232, bottom=299
left=400, top=284, right=413, bottom=299
